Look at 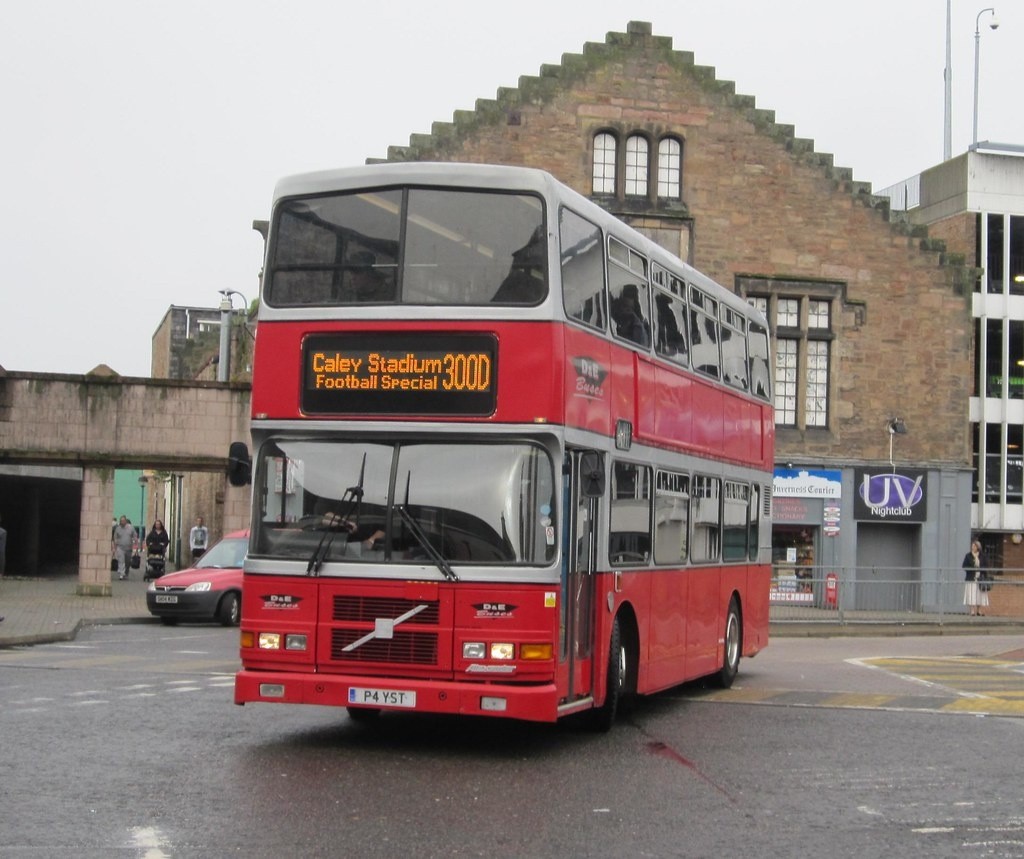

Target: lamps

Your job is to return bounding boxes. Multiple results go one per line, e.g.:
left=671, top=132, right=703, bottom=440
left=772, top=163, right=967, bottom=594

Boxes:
left=889, top=419, right=906, bottom=434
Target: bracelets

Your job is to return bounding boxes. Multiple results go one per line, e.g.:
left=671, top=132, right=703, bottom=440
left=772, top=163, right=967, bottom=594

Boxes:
left=368, top=538, right=374, bottom=544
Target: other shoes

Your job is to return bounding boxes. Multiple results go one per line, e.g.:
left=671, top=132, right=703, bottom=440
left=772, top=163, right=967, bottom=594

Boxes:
left=120, top=575, right=123, bottom=579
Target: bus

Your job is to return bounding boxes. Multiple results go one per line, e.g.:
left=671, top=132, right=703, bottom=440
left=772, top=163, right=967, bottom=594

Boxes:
left=233, top=161, right=774, bottom=733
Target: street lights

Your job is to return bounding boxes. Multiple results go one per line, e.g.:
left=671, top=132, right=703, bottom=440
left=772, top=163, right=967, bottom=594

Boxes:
left=137, top=477, right=148, bottom=552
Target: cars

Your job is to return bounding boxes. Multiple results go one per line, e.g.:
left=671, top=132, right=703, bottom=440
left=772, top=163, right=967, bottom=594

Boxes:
left=146, top=529, right=250, bottom=627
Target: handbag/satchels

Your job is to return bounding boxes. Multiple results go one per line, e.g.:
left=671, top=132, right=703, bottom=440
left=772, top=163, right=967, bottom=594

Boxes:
left=111, top=553, right=118, bottom=572
left=131, top=548, right=141, bottom=569
left=976, top=568, right=994, bottom=591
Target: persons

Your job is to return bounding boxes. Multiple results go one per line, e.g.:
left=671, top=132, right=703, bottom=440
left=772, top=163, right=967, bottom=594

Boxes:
left=960, top=541, right=990, bottom=616
left=146, top=519, right=169, bottom=554
left=111, top=515, right=138, bottom=579
left=313, top=478, right=386, bottom=550
left=189, top=517, right=208, bottom=558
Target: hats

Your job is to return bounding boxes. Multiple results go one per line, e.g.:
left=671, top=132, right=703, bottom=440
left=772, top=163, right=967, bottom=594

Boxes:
left=974, top=541, right=982, bottom=550
